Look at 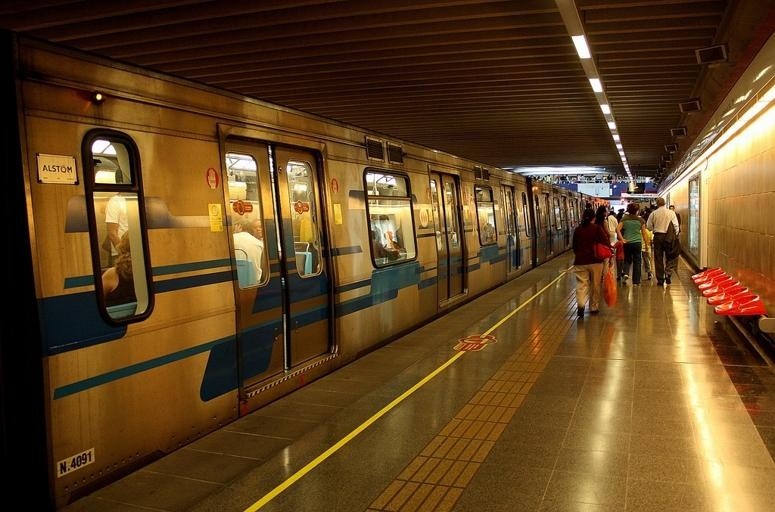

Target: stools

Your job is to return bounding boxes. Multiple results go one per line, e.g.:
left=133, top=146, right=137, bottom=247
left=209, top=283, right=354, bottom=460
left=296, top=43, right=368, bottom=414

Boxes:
left=691, top=267, right=765, bottom=318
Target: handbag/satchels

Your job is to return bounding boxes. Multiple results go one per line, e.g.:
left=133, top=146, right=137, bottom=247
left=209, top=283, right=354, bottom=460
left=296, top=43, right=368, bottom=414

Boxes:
left=603, top=271, right=617, bottom=307
left=614, top=240, right=624, bottom=261
left=591, top=243, right=613, bottom=259
left=665, top=239, right=680, bottom=260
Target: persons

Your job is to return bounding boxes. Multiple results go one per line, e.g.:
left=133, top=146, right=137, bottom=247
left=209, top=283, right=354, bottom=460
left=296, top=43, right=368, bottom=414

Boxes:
left=105, top=169, right=128, bottom=265
left=379, top=215, right=400, bottom=261
left=573, top=198, right=680, bottom=317
left=233, top=219, right=264, bottom=283
left=101, top=230, right=137, bottom=307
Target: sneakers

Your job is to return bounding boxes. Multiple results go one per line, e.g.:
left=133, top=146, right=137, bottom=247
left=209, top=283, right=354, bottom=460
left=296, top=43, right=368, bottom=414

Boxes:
left=648, top=272, right=653, bottom=280
left=577, top=307, right=585, bottom=316
left=622, top=275, right=629, bottom=284
left=590, top=309, right=599, bottom=314
left=633, top=282, right=639, bottom=288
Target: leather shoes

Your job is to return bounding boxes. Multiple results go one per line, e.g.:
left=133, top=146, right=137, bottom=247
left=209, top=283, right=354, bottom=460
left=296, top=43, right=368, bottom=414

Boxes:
left=657, top=279, right=664, bottom=285
left=666, top=277, right=671, bottom=285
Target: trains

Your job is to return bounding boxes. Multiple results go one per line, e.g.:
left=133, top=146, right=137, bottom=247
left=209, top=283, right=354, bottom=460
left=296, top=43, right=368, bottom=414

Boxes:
left=0, top=32, right=610, bottom=512
